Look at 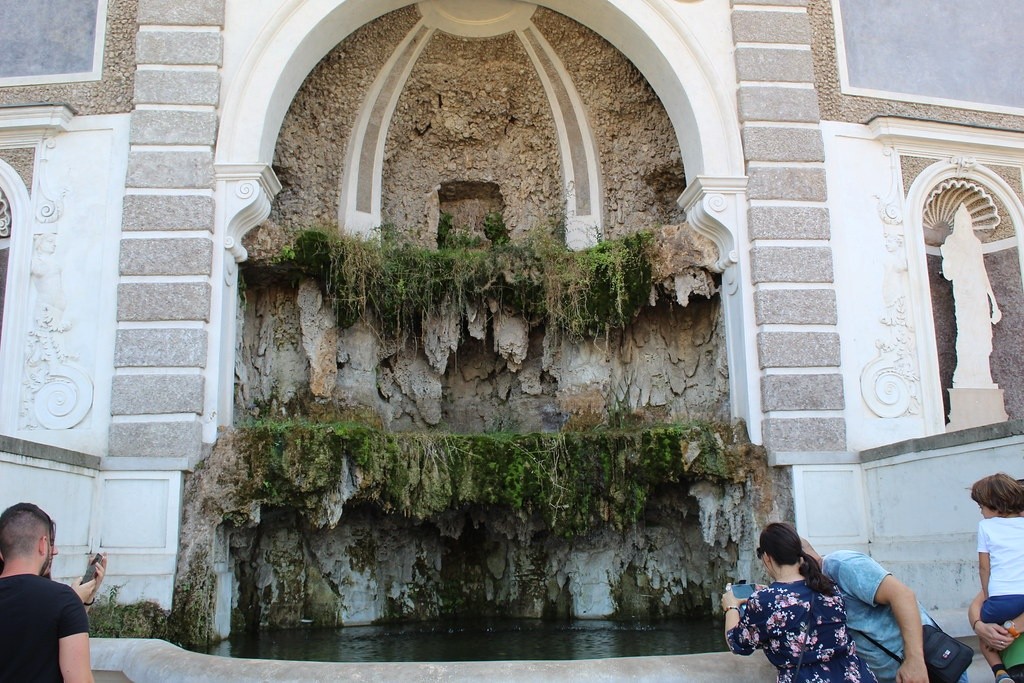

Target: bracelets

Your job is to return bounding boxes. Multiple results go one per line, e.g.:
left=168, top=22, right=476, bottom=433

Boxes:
left=972, top=619, right=982, bottom=632
left=723, top=606, right=741, bottom=616
left=84, top=598, right=95, bottom=606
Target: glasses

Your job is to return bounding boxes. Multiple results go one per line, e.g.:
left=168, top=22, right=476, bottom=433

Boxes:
left=757, top=550, right=763, bottom=560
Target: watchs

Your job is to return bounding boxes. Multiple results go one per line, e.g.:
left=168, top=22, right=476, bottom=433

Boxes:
left=1003, top=619, right=1020, bottom=639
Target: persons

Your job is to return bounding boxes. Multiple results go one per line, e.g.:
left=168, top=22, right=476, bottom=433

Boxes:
left=799, top=537, right=969, bottom=683
left=721, top=523, right=878, bottom=683
left=971, top=473, right=1024, bottom=683
left=0, top=504, right=107, bottom=683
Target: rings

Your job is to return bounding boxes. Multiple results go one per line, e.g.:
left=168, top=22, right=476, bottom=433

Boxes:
left=1001, top=640, right=1003, bottom=645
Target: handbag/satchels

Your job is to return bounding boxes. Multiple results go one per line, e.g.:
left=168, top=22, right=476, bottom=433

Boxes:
left=922, top=624, right=974, bottom=683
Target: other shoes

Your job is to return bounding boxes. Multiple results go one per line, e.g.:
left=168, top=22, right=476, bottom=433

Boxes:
left=996, top=674, right=1016, bottom=683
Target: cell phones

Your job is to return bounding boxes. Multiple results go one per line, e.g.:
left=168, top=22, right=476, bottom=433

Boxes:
left=731, top=583, right=758, bottom=597
left=80, top=553, right=105, bottom=584
